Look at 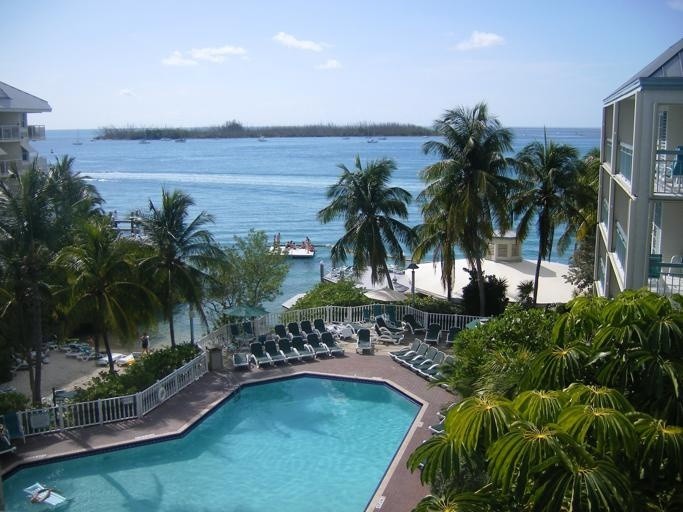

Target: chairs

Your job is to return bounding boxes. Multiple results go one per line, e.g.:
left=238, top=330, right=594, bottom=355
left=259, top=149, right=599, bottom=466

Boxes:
left=230, top=305, right=421, bottom=371
left=661, top=146, right=683, bottom=193
left=0, top=415, right=16, bottom=456
left=13, top=340, right=136, bottom=371
left=418, top=401, right=458, bottom=468
left=649, top=254, right=662, bottom=278
left=404, top=324, right=464, bottom=395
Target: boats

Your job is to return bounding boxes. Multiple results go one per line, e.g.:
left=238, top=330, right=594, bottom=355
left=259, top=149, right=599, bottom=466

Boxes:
left=267, top=245, right=315, bottom=257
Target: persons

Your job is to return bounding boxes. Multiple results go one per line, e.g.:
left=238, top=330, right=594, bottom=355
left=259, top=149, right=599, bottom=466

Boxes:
left=30, top=487, right=51, bottom=505
left=272, top=231, right=317, bottom=255
left=140, top=332, right=150, bottom=355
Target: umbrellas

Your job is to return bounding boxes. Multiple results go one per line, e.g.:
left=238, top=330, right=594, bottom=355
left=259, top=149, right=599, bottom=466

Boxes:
left=364, top=285, right=408, bottom=303
left=223, top=302, right=269, bottom=317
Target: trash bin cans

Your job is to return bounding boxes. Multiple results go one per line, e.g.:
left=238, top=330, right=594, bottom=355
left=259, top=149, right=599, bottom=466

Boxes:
left=208, top=348, right=222, bottom=370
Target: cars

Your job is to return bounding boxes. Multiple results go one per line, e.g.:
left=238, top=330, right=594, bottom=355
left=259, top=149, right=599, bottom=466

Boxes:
left=54, top=338, right=141, bottom=367
left=9, top=338, right=54, bottom=372
